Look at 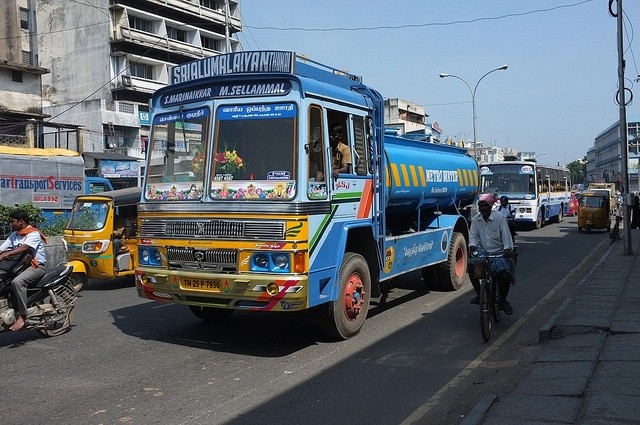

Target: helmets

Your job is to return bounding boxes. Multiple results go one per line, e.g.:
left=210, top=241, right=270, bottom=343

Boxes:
left=500, top=196, right=508, bottom=201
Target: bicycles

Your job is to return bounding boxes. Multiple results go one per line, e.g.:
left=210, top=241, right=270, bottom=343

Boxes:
left=468, top=243, right=511, bottom=342
left=608, top=215, right=623, bottom=246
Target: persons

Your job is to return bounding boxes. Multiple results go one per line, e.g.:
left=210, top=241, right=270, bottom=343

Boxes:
left=495, top=196, right=516, bottom=242
left=104, top=205, right=125, bottom=265
left=0, top=209, right=47, bottom=331
left=467, top=193, right=514, bottom=315
left=315, top=125, right=352, bottom=182
left=125, top=207, right=137, bottom=226
left=494, top=194, right=501, bottom=205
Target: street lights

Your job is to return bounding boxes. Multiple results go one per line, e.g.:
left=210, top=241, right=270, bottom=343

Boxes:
left=438, top=65, right=508, bottom=161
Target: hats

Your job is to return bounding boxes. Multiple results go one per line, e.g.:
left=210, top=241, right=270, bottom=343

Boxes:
left=478, top=193, right=498, bottom=207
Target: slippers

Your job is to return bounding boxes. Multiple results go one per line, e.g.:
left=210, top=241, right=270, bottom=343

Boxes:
left=503, top=301, right=512, bottom=315
left=469, top=297, right=478, bottom=303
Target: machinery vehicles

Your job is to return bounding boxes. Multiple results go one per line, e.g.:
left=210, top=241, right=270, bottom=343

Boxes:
left=131, top=51, right=480, bottom=341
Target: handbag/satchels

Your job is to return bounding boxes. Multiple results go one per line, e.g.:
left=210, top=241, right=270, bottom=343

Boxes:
left=0, top=243, right=32, bottom=275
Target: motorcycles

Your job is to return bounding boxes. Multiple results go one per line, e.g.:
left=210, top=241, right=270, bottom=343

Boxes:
left=0, top=259, right=78, bottom=335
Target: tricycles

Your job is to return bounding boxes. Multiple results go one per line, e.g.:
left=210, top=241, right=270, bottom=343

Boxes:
left=577, top=188, right=611, bottom=231
left=66, top=186, right=143, bottom=294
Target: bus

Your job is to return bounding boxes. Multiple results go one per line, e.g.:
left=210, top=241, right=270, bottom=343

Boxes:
left=480, top=152, right=572, bottom=229
